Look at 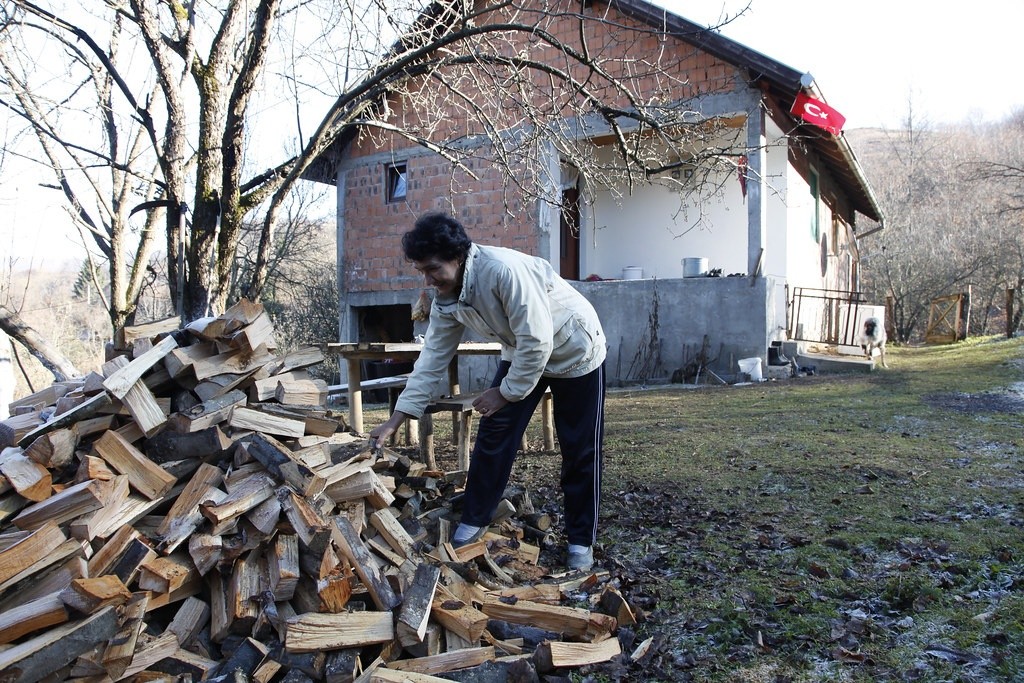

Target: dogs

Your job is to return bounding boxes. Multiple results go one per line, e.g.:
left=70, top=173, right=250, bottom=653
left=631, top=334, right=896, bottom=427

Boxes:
left=858, top=317, right=889, bottom=368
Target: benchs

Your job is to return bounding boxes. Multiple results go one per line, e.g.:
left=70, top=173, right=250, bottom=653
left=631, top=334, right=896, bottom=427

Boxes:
left=327, top=371, right=411, bottom=444
left=436, top=383, right=556, bottom=472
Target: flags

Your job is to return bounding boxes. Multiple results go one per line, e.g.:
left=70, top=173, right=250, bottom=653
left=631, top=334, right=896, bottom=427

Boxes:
left=791, top=92, right=846, bottom=137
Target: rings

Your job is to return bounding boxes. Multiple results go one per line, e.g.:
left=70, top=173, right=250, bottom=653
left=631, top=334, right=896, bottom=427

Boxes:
left=482, top=408, right=488, bottom=413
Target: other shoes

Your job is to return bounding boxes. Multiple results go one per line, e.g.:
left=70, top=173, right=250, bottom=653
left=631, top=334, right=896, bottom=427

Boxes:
left=567, top=542, right=594, bottom=571
left=450, top=522, right=490, bottom=548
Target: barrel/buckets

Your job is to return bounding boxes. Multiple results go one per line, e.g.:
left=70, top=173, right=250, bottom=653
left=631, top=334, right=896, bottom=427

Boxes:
left=682, top=257, right=709, bottom=278
left=622, top=265, right=643, bottom=279
left=738, top=357, right=762, bottom=381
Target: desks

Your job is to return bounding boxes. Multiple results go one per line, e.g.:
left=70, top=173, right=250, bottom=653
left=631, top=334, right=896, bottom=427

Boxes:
left=322, top=341, right=503, bottom=468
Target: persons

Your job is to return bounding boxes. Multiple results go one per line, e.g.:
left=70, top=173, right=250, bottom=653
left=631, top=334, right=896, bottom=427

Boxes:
left=371, top=209, right=607, bottom=573
